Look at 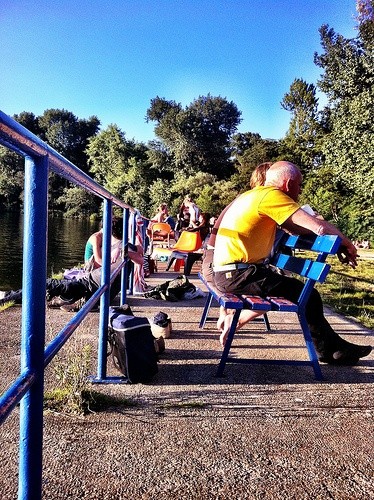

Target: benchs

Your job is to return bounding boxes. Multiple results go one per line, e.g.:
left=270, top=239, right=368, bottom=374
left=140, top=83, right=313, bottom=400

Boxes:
left=166, top=234, right=211, bottom=276
left=198, top=234, right=343, bottom=381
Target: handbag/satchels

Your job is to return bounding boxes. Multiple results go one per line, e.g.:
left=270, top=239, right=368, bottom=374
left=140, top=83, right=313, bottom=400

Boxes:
left=107, top=304, right=159, bottom=383
left=165, top=274, right=197, bottom=302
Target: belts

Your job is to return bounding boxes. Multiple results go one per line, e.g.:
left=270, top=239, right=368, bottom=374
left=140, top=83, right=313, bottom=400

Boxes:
left=212, top=263, right=249, bottom=272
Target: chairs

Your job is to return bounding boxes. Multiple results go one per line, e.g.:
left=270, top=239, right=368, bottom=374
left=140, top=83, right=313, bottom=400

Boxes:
left=150, top=223, right=172, bottom=272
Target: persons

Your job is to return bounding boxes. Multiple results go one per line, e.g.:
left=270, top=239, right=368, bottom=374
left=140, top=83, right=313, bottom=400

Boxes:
left=163, top=213, right=175, bottom=231
left=147, top=203, right=168, bottom=238
left=213, top=161, right=372, bottom=365
left=352, top=239, right=370, bottom=249
left=174, top=196, right=209, bottom=242
left=0, top=243, right=144, bottom=305
left=202, top=162, right=273, bottom=349
left=209, top=215, right=218, bottom=234
left=84, top=216, right=152, bottom=296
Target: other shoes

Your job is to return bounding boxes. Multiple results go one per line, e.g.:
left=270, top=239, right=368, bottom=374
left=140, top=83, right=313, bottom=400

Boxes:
left=47, top=297, right=74, bottom=309
left=318, top=337, right=372, bottom=365
left=60, top=296, right=98, bottom=312
